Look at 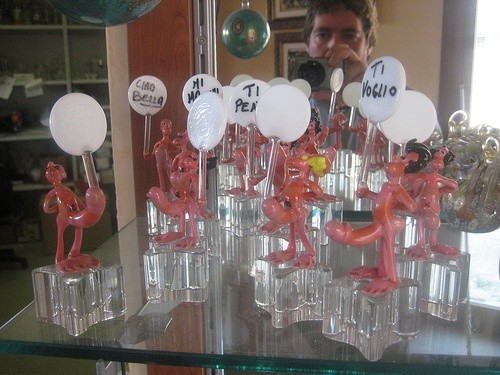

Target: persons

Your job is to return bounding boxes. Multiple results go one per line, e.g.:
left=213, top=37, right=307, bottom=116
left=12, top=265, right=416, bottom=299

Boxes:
left=302, top=0, right=443, bottom=157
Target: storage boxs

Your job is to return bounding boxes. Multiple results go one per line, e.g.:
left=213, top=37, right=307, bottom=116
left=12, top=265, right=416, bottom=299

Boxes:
left=38, top=190, right=112, bottom=254
left=15, top=217, right=42, bottom=242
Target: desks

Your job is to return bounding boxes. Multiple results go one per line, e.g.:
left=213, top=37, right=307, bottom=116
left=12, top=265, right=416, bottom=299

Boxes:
left=0, top=207, right=500, bottom=375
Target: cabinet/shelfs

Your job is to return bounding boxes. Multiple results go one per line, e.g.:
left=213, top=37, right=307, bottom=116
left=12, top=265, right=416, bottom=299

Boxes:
left=0, top=0, right=115, bottom=191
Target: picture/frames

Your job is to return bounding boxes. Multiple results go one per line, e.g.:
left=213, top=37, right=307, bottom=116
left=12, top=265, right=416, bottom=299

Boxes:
left=266, top=0, right=311, bottom=30
left=273, top=31, right=307, bottom=80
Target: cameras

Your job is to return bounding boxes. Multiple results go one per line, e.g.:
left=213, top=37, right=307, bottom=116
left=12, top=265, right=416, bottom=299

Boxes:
left=288, top=55, right=344, bottom=91
